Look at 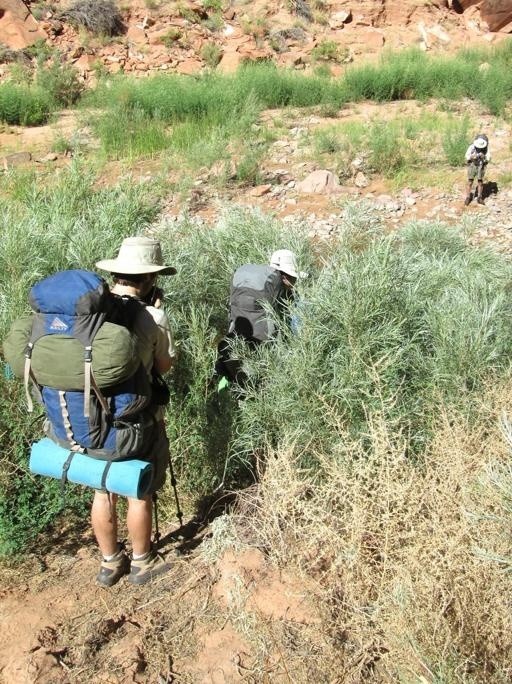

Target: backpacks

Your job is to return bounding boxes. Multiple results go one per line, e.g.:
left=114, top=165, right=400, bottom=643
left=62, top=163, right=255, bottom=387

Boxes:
left=473, top=135, right=488, bottom=154
left=3, top=271, right=158, bottom=462
left=229, top=263, right=282, bottom=343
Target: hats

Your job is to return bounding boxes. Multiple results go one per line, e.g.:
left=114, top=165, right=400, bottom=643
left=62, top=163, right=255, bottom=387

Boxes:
left=474, top=138, right=487, bottom=149
left=96, top=237, right=177, bottom=276
left=271, top=249, right=310, bottom=279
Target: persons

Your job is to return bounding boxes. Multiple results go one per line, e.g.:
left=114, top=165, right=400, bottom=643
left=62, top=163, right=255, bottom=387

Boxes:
left=463, top=137, right=491, bottom=206
left=228, top=248, right=309, bottom=346
left=40, top=235, right=179, bottom=588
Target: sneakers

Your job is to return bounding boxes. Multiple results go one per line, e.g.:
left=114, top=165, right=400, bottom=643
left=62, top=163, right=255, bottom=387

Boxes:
left=465, top=197, right=471, bottom=206
left=96, top=552, right=130, bottom=588
left=129, top=546, right=180, bottom=586
left=478, top=197, right=485, bottom=205
